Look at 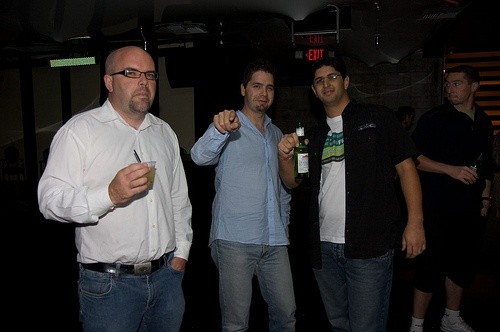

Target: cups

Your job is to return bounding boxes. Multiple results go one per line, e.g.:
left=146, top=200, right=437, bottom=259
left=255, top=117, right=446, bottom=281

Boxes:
left=141, top=161, right=156, bottom=190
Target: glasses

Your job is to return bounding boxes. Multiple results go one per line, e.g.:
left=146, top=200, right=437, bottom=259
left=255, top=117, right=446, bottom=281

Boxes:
left=109, top=67, right=159, bottom=82
left=313, top=72, right=343, bottom=85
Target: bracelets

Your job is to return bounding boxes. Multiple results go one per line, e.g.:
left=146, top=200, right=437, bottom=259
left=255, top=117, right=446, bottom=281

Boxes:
left=279, top=155, right=293, bottom=160
left=482, top=197, right=491, bottom=201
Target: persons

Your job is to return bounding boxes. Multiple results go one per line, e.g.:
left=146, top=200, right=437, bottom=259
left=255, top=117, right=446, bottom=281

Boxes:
left=190, top=60, right=296, bottom=332
left=395, top=105, right=415, bottom=129
left=410, top=66, right=493, bottom=332
left=37, top=46, right=194, bottom=332
left=278, top=57, right=425, bottom=332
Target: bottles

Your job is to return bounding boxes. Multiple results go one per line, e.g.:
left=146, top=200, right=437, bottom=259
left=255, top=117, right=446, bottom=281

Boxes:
left=462, top=151, right=484, bottom=185
left=294, top=121, right=310, bottom=184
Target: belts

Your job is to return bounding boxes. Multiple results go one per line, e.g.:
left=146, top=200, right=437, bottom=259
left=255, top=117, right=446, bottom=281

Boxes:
left=82, top=250, right=175, bottom=275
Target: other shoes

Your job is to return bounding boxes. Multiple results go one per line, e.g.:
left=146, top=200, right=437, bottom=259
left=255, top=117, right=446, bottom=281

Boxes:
left=409, top=323, right=424, bottom=332
left=440, top=314, right=476, bottom=332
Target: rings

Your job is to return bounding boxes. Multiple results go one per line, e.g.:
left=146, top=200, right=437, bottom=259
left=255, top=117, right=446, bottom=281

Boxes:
left=418, top=248, right=422, bottom=250
